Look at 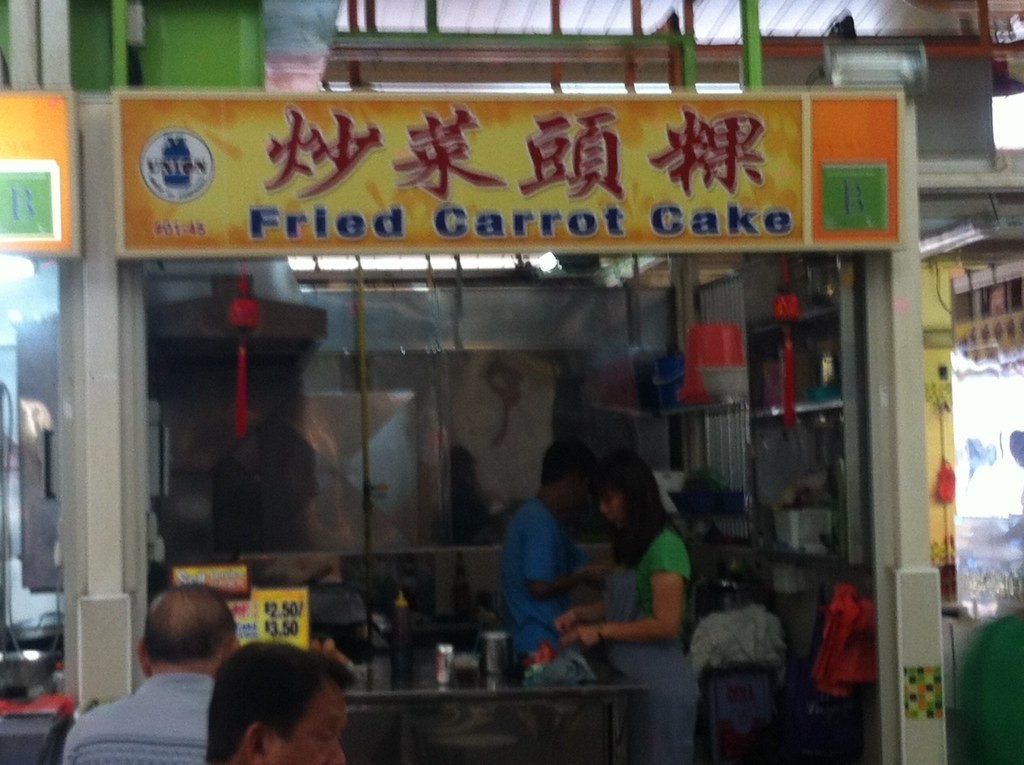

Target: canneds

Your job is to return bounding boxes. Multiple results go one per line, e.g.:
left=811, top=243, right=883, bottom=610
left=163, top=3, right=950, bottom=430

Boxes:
left=435, top=644, right=455, bottom=684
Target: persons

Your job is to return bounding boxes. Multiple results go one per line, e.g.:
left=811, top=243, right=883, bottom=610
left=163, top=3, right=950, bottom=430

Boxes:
left=451, top=451, right=499, bottom=546
left=202, top=637, right=357, bottom=765
left=61, top=582, right=240, bottom=765
left=689, top=570, right=790, bottom=686
left=502, top=434, right=704, bottom=765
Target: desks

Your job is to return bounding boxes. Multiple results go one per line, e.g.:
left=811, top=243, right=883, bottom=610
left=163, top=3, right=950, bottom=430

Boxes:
left=341, top=652, right=637, bottom=765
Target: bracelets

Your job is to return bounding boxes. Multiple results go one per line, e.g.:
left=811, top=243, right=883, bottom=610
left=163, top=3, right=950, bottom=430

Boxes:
left=594, top=620, right=603, bottom=641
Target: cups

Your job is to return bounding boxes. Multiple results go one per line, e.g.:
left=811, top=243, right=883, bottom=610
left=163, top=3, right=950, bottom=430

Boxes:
left=436, top=644, right=454, bottom=684
left=478, top=632, right=514, bottom=675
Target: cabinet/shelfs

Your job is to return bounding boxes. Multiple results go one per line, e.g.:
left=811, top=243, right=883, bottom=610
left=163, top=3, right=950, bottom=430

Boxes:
left=747, top=289, right=875, bottom=567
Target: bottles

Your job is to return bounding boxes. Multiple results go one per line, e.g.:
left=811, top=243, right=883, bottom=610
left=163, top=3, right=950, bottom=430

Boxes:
left=393, top=591, right=411, bottom=644
